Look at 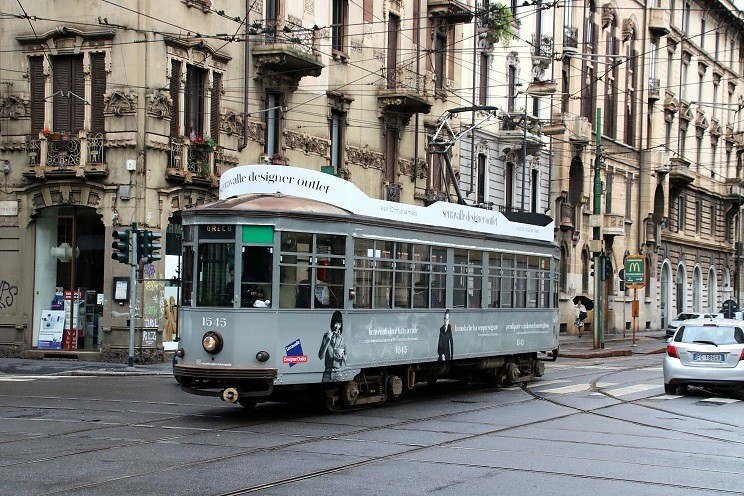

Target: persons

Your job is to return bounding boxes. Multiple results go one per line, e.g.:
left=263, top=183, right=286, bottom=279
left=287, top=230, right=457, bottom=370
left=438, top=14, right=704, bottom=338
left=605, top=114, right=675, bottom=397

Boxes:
left=437, top=309, right=453, bottom=362
left=573, top=299, right=588, bottom=338
left=318, top=309, right=348, bottom=382
left=222, top=265, right=264, bottom=307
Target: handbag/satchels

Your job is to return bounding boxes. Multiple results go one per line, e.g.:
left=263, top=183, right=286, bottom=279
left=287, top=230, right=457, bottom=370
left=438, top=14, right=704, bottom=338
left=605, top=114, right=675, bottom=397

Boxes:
left=579, top=312, right=588, bottom=321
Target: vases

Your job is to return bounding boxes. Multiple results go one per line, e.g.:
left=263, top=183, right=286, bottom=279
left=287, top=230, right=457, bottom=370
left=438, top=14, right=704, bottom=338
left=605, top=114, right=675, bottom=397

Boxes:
left=338, top=171, right=349, bottom=177
left=47, top=134, right=62, bottom=141
left=194, top=142, right=205, bottom=152
left=272, top=158, right=285, bottom=165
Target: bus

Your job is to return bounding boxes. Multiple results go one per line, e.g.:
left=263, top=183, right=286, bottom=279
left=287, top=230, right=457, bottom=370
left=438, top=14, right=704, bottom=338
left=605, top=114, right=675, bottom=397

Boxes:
left=172, top=106, right=561, bottom=413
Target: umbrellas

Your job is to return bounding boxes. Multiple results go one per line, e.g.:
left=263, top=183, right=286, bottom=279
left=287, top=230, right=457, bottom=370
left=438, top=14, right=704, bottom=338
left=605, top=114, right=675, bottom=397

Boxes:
left=573, top=296, right=594, bottom=311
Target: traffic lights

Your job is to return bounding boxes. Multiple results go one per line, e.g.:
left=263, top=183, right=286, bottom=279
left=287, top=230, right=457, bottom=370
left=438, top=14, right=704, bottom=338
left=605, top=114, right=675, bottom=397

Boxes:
left=590, top=257, right=595, bottom=277
left=111, top=229, right=130, bottom=264
left=600, top=255, right=610, bottom=279
left=147, top=231, right=162, bottom=263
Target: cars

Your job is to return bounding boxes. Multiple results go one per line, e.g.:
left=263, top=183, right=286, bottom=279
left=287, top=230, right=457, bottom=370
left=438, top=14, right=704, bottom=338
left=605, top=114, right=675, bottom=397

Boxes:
left=666, top=312, right=744, bottom=336
left=662, top=318, right=744, bottom=395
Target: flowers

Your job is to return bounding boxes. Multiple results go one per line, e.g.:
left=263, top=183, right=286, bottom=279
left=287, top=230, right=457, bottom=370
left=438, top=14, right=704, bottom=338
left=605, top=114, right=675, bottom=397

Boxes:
left=272, top=152, right=285, bottom=161
left=337, top=167, right=351, bottom=175
left=41, top=128, right=61, bottom=137
left=192, top=137, right=206, bottom=146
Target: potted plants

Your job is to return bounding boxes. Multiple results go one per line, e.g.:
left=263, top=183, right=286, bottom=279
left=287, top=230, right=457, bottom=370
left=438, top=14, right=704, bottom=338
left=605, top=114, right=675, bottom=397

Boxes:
left=204, top=137, right=218, bottom=153
left=61, top=131, right=71, bottom=140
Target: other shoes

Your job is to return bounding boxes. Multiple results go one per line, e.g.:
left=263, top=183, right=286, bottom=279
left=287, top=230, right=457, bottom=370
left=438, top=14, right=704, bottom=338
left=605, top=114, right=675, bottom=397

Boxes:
left=579, top=334, right=582, bottom=338
